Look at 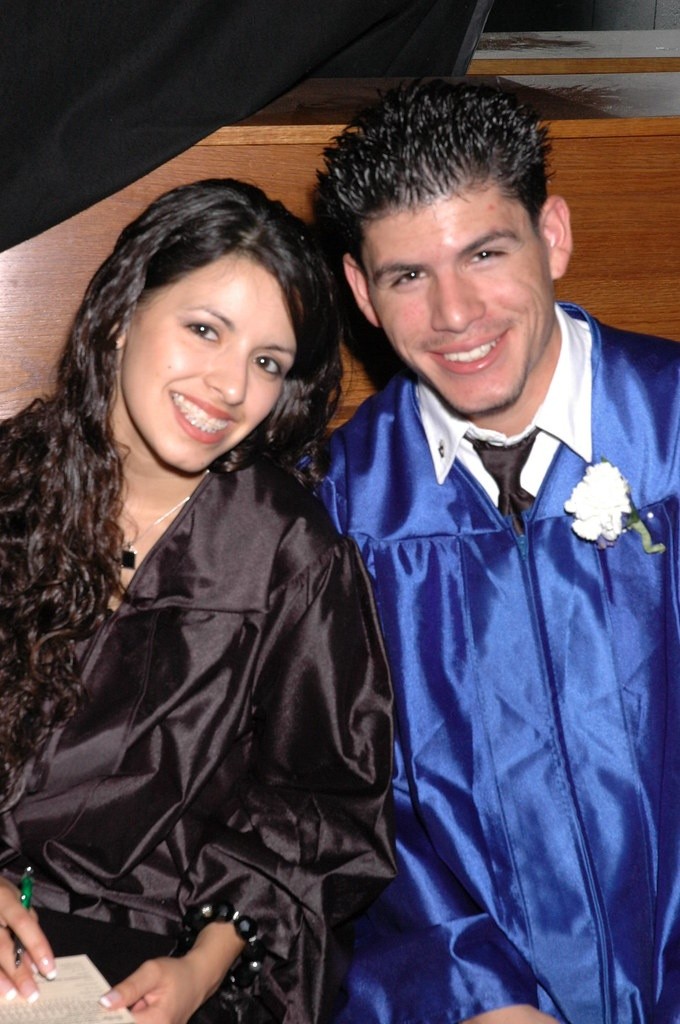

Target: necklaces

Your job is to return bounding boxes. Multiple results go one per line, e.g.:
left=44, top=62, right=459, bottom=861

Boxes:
left=118, top=469, right=211, bottom=569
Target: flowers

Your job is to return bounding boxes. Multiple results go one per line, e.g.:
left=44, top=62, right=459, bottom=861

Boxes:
left=563, top=452, right=667, bottom=557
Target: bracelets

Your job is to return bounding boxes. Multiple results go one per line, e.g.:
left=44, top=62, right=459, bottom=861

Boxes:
left=182, top=902, right=262, bottom=1003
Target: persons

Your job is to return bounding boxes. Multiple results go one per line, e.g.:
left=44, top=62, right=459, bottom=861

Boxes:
left=309, top=76, right=680, bottom=1024
left=1, top=78, right=396, bottom=1024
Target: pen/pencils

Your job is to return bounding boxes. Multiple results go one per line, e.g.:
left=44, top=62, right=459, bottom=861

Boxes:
left=14, top=866, right=34, bottom=969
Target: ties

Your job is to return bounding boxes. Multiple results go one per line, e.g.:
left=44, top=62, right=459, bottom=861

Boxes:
left=464, top=428, right=541, bottom=535
